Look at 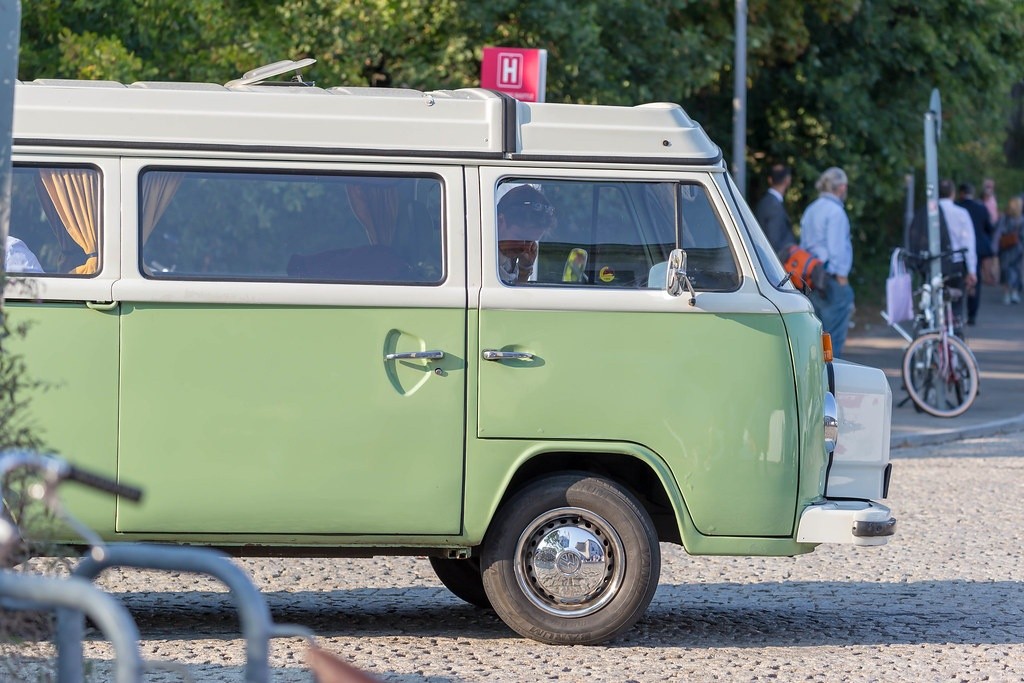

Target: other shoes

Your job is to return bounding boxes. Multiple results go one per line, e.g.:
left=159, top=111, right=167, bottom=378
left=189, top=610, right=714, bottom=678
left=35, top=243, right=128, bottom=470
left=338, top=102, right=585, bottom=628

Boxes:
left=966, top=316, right=975, bottom=325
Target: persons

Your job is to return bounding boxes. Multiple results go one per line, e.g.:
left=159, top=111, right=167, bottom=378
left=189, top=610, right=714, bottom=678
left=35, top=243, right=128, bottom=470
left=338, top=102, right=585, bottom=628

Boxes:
left=497, top=185, right=554, bottom=283
left=755, top=164, right=855, bottom=359
left=909, top=179, right=1024, bottom=342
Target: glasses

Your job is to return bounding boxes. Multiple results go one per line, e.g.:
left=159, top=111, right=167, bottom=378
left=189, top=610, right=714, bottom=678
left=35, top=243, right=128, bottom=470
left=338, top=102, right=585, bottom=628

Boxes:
left=512, top=201, right=557, bottom=217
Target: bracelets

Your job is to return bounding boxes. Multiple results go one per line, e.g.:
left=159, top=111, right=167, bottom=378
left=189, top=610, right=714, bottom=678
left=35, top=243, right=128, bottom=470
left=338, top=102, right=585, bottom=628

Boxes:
left=518, top=262, right=533, bottom=277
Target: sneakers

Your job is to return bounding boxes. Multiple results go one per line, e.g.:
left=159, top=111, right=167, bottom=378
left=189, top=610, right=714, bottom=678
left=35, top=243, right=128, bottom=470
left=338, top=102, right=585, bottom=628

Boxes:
left=1002, top=291, right=1022, bottom=306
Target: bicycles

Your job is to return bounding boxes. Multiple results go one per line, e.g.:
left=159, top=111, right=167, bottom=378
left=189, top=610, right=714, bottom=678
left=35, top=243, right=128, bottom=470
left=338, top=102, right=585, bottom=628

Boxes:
left=883, top=248, right=981, bottom=420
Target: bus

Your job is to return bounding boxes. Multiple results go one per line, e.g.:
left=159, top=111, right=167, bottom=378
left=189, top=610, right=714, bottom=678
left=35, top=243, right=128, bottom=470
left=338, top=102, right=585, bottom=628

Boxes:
left=0, top=77, right=894, bottom=644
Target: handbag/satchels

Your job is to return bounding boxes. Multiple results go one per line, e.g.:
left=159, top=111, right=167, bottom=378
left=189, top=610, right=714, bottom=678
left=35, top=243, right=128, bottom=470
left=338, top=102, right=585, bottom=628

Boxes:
left=886, top=248, right=915, bottom=326
left=999, top=231, right=1018, bottom=250
